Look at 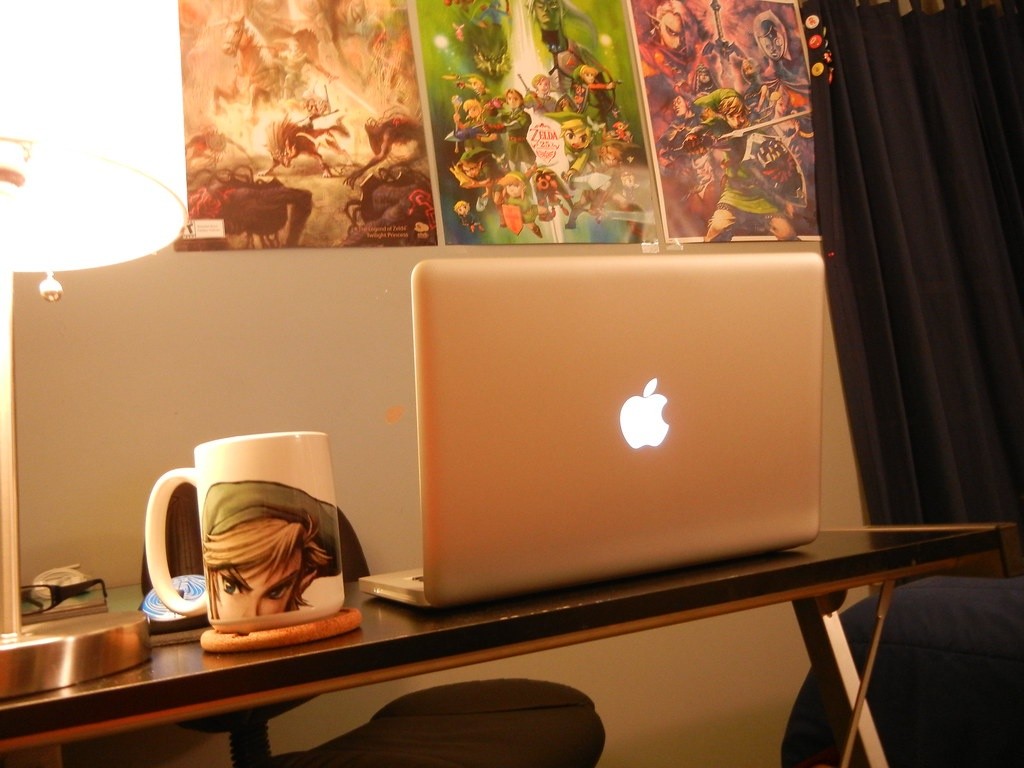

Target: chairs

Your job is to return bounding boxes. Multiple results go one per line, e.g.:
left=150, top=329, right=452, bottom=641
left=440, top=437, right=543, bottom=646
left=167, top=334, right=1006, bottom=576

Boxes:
left=142, top=483, right=606, bottom=768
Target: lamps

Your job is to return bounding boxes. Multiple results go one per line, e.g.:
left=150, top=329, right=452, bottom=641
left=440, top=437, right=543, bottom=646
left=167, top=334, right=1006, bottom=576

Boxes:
left=1, top=0, right=191, bottom=704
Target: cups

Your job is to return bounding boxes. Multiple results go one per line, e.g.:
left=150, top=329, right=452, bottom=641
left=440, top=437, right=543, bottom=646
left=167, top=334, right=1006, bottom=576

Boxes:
left=144, top=429, right=345, bottom=635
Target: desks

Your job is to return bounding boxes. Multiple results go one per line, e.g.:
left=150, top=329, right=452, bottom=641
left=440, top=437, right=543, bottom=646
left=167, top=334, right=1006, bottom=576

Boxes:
left=2, top=524, right=1024, bottom=768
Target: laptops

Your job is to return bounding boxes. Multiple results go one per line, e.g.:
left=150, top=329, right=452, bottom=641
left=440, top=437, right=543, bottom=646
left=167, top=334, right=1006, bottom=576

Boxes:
left=357, top=254, right=825, bottom=610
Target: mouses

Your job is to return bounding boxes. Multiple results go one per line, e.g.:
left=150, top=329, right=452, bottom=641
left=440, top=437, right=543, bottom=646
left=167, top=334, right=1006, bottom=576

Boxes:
left=137, top=576, right=213, bottom=635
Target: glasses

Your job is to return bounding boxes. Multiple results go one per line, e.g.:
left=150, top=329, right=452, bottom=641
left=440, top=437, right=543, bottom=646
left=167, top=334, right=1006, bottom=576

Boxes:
left=19, top=577, right=109, bottom=618
